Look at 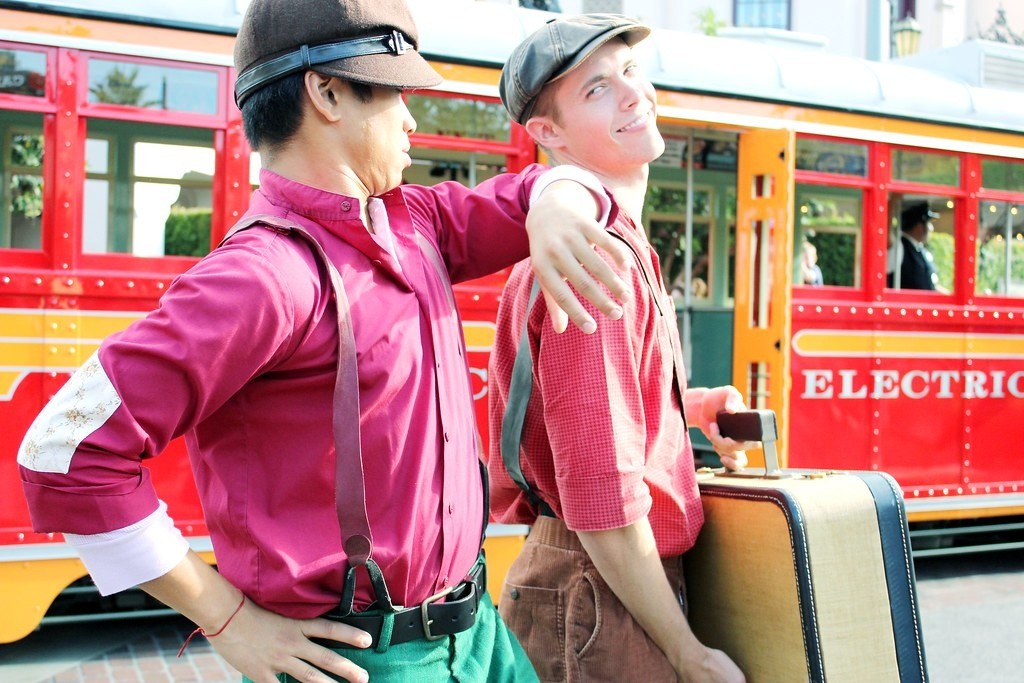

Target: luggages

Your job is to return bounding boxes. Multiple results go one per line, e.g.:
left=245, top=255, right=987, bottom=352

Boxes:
left=691, top=410, right=929, bottom=683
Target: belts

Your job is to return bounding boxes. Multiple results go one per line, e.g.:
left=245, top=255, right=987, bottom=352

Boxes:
left=311, top=549, right=485, bottom=649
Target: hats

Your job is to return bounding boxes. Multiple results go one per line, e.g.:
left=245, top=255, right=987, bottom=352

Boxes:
left=901, top=204, right=940, bottom=226
left=234, top=0, right=443, bottom=108
left=499, top=15, right=651, bottom=126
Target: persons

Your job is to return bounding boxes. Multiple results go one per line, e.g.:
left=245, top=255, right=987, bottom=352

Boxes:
left=888, top=201, right=941, bottom=289
left=802, top=244, right=823, bottom=284
left=17, top=0, right=633, bottom=683
left=498, top=14, right=748, bottom=683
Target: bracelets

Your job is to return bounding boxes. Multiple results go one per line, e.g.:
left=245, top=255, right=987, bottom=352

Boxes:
left=177, top=592, right=245, bottom=657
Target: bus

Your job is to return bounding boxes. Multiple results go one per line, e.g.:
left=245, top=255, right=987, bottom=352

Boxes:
left=0, top=0, right=1024, bottom=648
